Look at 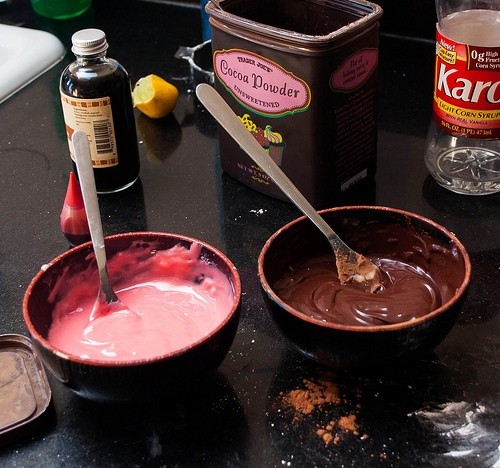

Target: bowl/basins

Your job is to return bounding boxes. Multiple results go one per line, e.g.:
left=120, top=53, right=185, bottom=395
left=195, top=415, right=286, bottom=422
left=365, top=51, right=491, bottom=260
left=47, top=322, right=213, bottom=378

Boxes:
left=257, top=205, right=471, bottom=356
left=21, top=232, right=242, bottom=404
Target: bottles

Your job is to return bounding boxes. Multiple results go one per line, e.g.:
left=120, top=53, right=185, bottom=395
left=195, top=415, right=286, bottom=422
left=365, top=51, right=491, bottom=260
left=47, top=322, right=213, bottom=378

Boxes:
left=60, top=29, right=141, bottom=193
left=424, top=0, right=500, bottom=195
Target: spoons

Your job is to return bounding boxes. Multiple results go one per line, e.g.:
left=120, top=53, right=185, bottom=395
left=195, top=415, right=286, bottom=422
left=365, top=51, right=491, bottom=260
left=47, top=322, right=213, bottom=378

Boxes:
left=196, top=84, right=393, bottom=294
left=71, top=131, right=141, bottom=336
